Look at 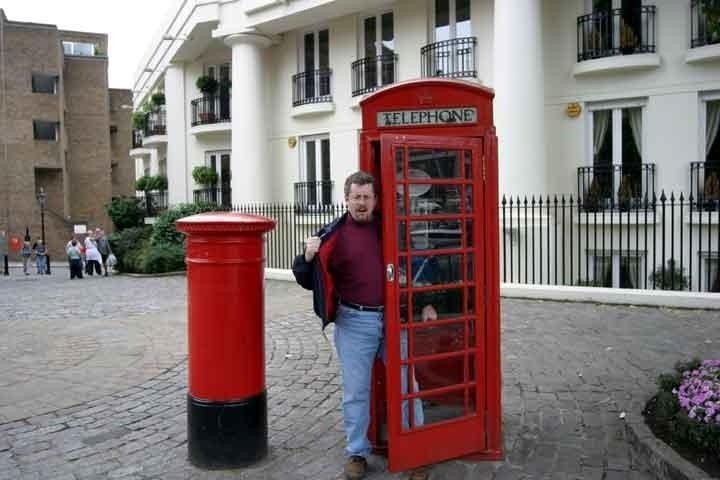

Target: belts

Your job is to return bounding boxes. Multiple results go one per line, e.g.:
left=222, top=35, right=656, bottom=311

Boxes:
left=340, top=298, right=384, bottom=312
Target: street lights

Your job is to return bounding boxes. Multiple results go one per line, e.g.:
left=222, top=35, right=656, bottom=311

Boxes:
left=37, top=186, right=50, bottom=274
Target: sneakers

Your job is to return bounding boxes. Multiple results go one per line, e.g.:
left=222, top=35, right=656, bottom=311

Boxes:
left=344, top=455, right=367, bottom=479
left=409, top=465, right=432, bottom=479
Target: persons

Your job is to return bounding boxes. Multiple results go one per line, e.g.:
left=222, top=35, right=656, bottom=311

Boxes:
left=65, top=227, right=112, bottom=279
left=291, top=171, right=439, bottom=479
left=21, top=236, right=48, bottom=276
